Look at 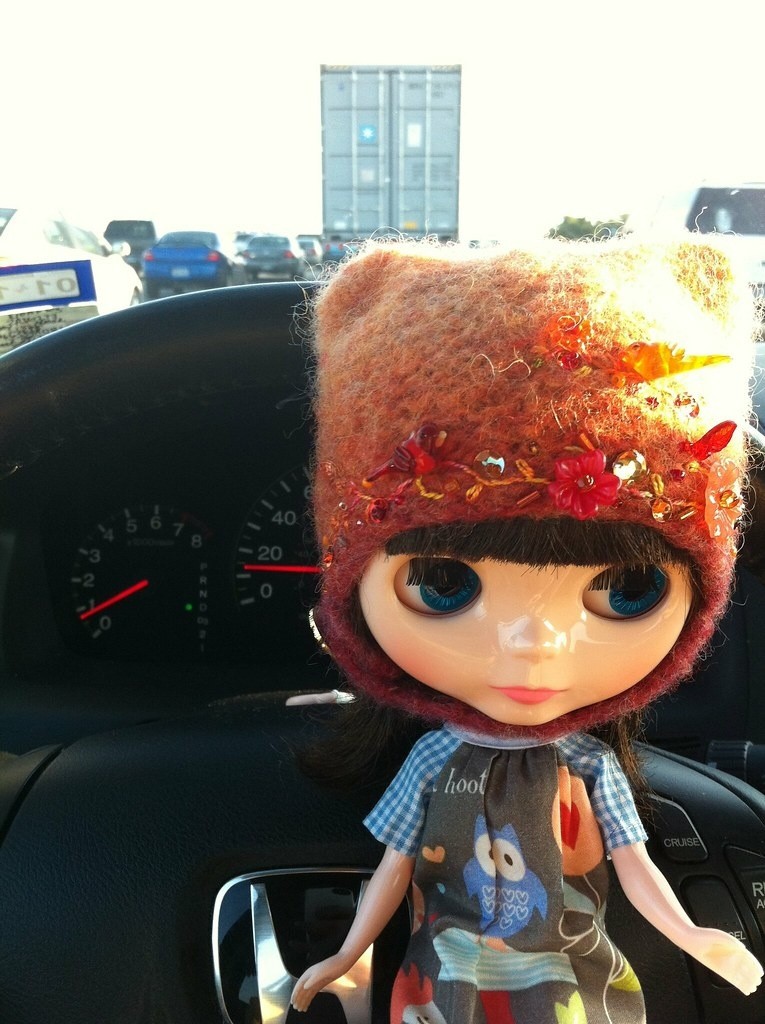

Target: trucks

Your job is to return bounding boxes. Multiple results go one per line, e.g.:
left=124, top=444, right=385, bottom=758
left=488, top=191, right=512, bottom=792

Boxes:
left=320, top=61, right=463, bottom=244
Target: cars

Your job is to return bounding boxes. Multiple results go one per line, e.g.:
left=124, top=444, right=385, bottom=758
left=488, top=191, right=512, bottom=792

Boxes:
left=297, top=238, right=323, bottom=270
left=321, top=241, right=349, bottom=265
left=0, top=206, right=144, bottom=322
left=143, top=229, right=233, bottom=303
left=243, top=236, right=307, bottom=279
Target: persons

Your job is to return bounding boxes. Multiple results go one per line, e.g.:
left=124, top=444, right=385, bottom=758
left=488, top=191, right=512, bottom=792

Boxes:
left=290, top=234, right=765, bottom=1024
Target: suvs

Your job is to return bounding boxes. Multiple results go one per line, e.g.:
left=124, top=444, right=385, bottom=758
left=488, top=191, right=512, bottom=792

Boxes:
left=103, top=218, right=157, bottom=279
